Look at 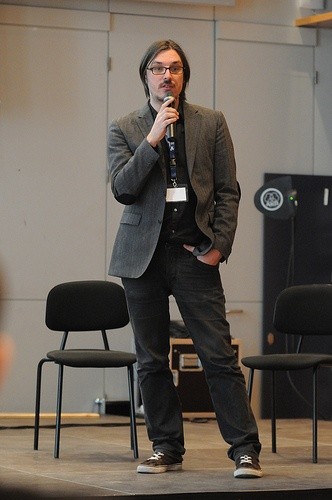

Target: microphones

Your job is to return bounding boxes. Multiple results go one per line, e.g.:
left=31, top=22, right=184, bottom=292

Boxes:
left=164, top=93, right=176, bottom=141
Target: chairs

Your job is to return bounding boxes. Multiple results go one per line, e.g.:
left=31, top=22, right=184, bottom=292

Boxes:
left=33, top=280, right=140, bottom=461
left=240, top=284, right=332, bottom=464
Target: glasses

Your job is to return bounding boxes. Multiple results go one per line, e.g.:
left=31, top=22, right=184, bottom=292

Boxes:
left=147, top=65, right=183, bottom=74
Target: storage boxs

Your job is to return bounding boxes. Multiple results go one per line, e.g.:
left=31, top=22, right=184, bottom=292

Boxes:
left=168, top=336, right=243, bottom=422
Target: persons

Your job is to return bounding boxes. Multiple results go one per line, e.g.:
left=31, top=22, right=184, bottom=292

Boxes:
left=104, top=39, right=266, bottom=479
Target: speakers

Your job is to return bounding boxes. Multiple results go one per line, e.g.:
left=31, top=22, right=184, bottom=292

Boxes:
left=259, top=173, right=332, bottom=421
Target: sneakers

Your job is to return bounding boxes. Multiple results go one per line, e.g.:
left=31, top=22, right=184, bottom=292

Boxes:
left=233, top=455, right=264, bottom=478
left=135, top=451, right=183, bottom=475
